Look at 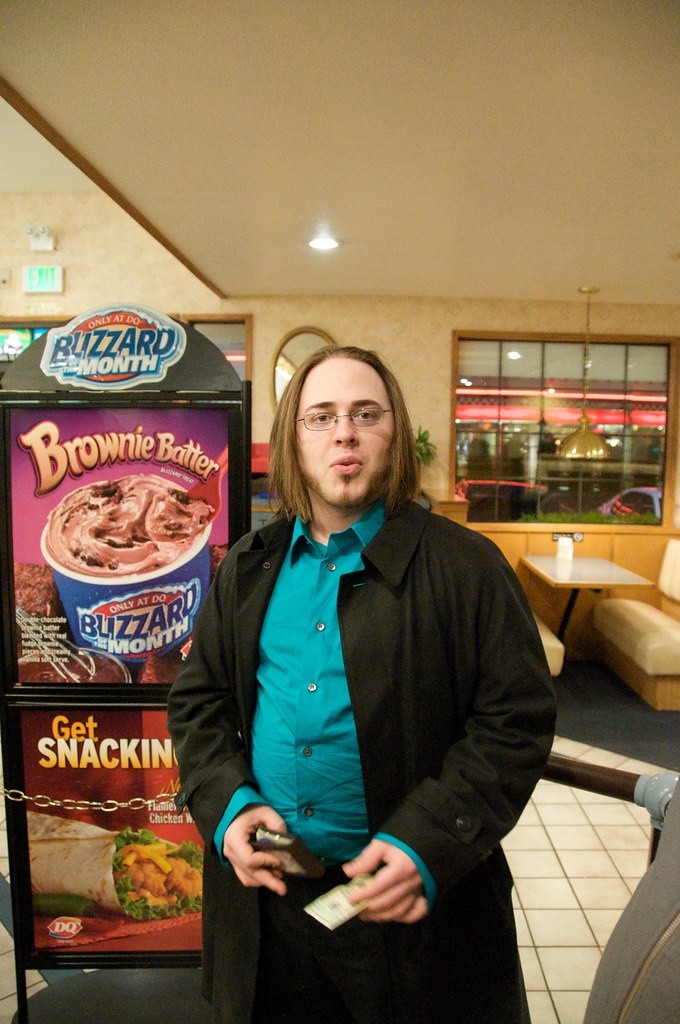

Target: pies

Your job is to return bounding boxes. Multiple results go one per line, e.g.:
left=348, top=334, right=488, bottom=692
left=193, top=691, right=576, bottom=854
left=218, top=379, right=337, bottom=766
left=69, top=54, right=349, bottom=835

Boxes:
left=28, top=808, right=206, bottom=916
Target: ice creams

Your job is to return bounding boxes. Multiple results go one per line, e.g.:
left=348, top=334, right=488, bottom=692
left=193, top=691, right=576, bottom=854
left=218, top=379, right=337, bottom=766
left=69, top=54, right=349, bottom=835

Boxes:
left=44, top=475, right=213, bottom=652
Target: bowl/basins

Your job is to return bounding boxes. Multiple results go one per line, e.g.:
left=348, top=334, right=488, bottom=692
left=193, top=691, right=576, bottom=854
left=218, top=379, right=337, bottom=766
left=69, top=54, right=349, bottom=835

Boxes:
left=40, top=522, right=212, bottom=660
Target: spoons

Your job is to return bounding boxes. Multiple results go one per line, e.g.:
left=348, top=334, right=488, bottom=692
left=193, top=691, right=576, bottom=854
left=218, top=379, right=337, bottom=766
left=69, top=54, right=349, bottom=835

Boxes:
left=16, top=606, right=95, bottom=681
left=184, top=445, right=229, bottom=530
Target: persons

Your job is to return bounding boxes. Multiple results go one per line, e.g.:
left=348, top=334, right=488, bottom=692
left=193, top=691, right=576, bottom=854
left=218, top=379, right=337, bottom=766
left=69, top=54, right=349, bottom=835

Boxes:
left=166, top=348, right=557, bottom=1024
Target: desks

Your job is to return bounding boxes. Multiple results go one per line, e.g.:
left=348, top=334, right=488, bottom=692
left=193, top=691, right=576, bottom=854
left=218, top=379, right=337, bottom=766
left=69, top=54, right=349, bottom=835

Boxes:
left=522, top=554, right=658, bottom=637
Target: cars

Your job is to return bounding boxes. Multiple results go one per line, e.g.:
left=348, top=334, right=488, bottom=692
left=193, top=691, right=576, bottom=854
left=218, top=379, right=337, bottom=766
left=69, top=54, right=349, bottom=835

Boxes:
left=598, top=485, right=661, bottom=519
left=455, top=478, right=613, bottom=523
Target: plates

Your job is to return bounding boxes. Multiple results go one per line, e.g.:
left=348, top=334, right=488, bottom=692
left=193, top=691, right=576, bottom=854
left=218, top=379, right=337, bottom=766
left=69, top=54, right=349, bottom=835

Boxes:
left=18, top=647, right=133, bottom=683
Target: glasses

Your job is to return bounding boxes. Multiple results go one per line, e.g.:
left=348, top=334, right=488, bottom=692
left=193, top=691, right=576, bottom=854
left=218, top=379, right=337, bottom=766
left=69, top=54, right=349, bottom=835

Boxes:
left=295, top=408, right=394, bottom=430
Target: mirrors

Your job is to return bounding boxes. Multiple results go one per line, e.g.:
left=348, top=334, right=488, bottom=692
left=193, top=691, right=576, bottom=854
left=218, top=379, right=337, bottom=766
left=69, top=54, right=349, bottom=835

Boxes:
left=270, top=326, right=335, bottom=416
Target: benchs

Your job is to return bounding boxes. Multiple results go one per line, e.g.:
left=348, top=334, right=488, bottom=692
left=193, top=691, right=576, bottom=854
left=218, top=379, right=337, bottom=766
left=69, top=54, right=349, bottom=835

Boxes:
left=591, top=538, right=680, bottom=714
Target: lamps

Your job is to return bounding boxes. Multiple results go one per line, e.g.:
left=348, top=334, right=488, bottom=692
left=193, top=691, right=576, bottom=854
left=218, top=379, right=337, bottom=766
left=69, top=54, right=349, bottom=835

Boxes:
left=26, top=225, right=55, bottom=251
left=557, top=287, right=612, bottom=458
